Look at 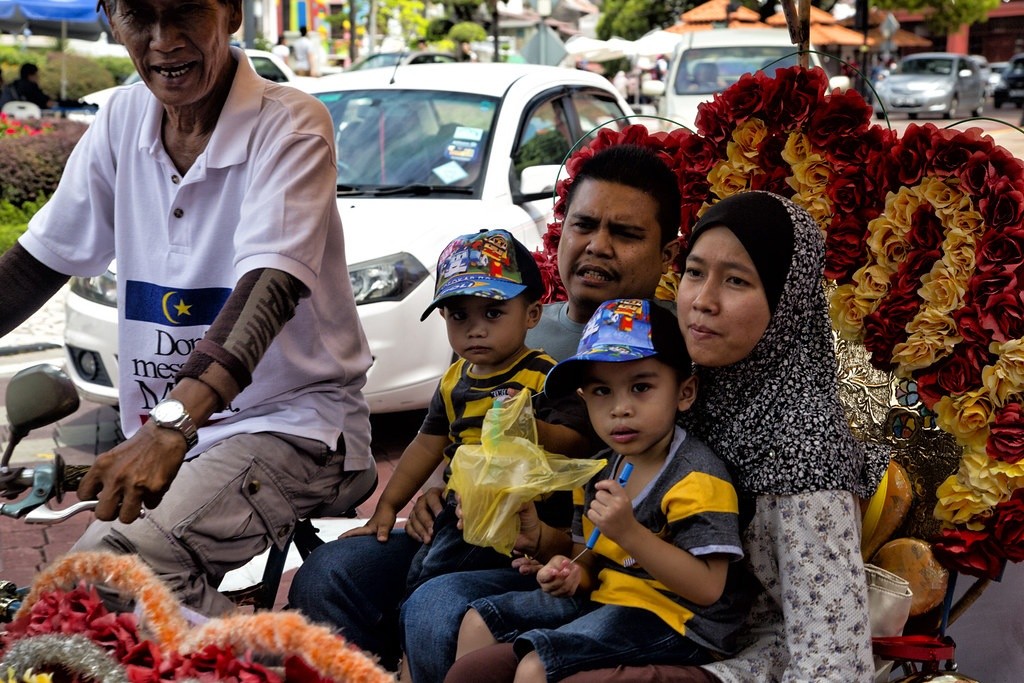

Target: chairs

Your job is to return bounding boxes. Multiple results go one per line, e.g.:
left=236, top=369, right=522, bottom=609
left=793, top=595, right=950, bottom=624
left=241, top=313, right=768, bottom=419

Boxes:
left=2, top=101, right=41, bottom=120
left=1, top=83, right=22, bottom=101
left=687, top=62, right=722, bottom=93
left=351, top=107, right=427, bottom=188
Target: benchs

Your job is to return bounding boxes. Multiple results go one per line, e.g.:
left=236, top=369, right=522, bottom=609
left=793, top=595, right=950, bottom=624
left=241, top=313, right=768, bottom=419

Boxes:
left=523, top=50, right=1023, bottom=683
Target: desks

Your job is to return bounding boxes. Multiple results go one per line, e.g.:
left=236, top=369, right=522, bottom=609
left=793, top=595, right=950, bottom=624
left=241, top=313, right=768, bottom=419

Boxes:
left=41, top=106, right=98, bottom=119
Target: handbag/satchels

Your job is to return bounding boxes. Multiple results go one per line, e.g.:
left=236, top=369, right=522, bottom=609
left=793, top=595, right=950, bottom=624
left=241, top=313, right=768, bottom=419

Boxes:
left=447, top=387, right=608, bottom=558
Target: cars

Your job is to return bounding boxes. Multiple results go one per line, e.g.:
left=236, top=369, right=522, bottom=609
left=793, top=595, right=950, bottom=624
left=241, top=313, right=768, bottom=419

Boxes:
left=871, top=52, right=990, bottom=121
left=652, top=27, right=851, bottom=136
left=61, top=46, right=646, bottom=418
left=973, top=52, right=1024, bottom=111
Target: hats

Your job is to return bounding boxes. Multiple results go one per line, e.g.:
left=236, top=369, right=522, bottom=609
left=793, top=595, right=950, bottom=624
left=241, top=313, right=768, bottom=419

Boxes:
left=420, top=227, right=548, bottom=323
left=544, top=298, right=692, bottom=406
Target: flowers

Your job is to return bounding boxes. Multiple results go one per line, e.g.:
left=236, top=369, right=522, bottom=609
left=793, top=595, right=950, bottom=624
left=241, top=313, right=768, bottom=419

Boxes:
left=533, top=66, right=1023, bottom=579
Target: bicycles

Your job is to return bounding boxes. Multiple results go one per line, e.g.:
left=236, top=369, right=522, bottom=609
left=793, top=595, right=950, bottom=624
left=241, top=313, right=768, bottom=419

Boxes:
left=0, top=361, right=380, bottom=625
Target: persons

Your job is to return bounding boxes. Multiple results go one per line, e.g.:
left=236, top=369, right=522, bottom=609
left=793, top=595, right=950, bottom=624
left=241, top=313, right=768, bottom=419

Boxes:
left=457, top=296, right=743, bottom=683
left=271, top=21, right=480, bottom=72
left=274, top=143, right=691, bottom=683
left=338, top=229, right=604, bottom=683
left=443, top=190, right=878, bottom=683
left=574, top=53, right=668, bottom=117
left=842, top=53, right=890, bottom=103
left=0, top=0, right=379, bottom=624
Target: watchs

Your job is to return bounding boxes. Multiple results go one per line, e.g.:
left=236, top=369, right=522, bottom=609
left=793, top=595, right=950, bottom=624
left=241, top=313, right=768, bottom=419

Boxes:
left=148, top=397, right=200, bottom=453
